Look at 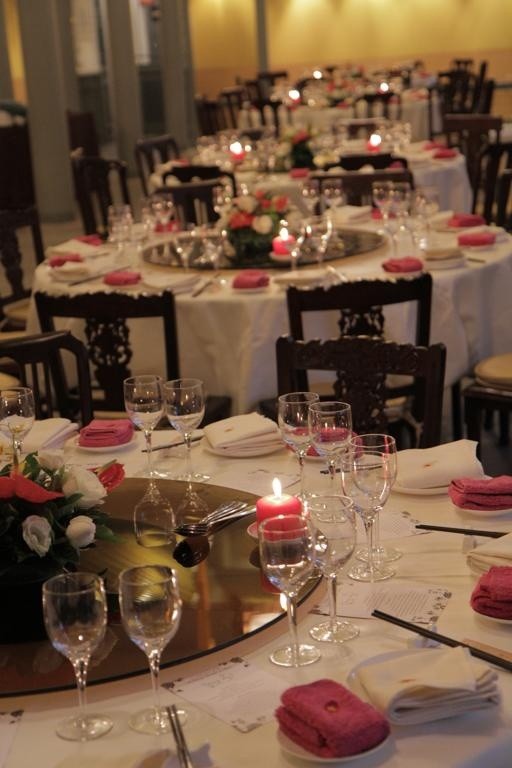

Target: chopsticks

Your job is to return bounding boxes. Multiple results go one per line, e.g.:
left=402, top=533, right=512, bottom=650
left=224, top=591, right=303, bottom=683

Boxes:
left=163, top=704, right=196, bottom=768
left=371, top=610, right=512, bottom=674
left=413, top=522, right=510, bottom=540
left=141, top=435, right=203, bottom=453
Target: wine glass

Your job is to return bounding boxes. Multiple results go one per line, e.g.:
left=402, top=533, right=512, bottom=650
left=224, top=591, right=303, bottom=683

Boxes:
left=46, top=174, right=508, bottom=293
left=0, top=387, right=35, bottom=477
left=128, top=479, right=177, bottom=547
left=38, top=572, right=115, bottom=743
left=300, top=493, right=363, bottom=642
left=177, top=483, right=208, bottom=526
left=122, top=374, right=171, bottom=479
left=184, top=66, right=464, bottom=173
left=117, top=564, right=189, bottom=735
left=273, top=387, right=406, bottom=583
left=256, top=514, right=321, bottom=667
left=159, top=377, right=213, bottom=484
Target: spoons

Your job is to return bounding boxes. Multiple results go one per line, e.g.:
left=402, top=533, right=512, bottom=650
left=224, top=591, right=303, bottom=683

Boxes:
left=173, top=498, right=257, bottom=538
left=171, top=535, right=215, bottom=568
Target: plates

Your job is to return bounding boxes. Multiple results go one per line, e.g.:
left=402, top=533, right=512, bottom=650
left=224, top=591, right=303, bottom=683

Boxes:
left=448, top=496, right=512, bottom=518
left=470, top=602, right=512, bottom=626
left=272, top=712, right=392, bottom=762
left=392, top=480, right=453, bottom=497
left=66, top=434, right=137, bottom=453
left=464, top=558, right=489, bottom=581
left=344, top=648, right=448, bottom=709
left=198, top=434, right=287, bottom=460
left=247, top=520, right=312, bottom=547
left=248, top=543, right=303, bottom=568
